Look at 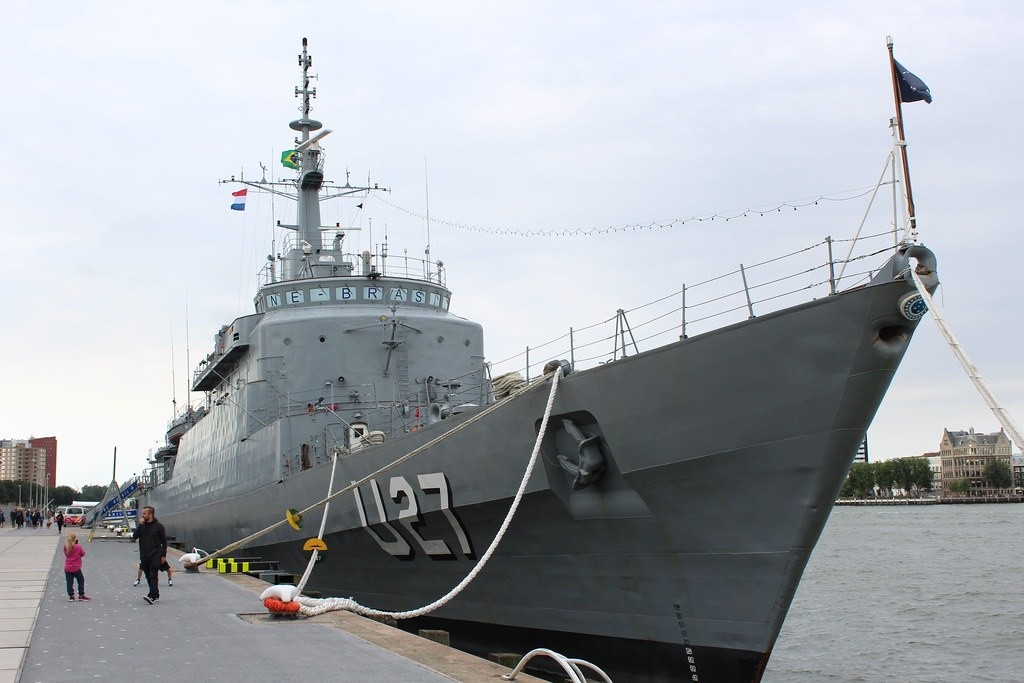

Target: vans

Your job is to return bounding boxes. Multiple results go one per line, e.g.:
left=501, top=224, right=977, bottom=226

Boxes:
left=55, top=506, right=84, bottom=525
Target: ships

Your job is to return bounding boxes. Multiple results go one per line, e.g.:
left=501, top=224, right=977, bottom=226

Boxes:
left=139, top=33, right=939, bottom=681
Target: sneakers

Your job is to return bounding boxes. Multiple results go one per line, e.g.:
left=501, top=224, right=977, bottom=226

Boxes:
left=68, top=595, right=76, bottom=602
left=143, top=593, right=159, bottom=605
left=77, top=594, right=91, bottom=601
left=168, top=578, right=173, bottom=586
left=133, top=579, right=141, bottom=587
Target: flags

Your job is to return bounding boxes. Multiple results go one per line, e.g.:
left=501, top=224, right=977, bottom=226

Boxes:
left=894, top=59, right=932, bottom=104
left=230, top=189, right=247, bottom=210
left=281, top=150, right=299, bottom=171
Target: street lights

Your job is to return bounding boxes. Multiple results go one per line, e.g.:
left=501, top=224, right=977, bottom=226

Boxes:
left=19, top=458, right=51, bottom=520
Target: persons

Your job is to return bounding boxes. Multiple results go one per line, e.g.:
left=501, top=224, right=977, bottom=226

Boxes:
left=10, top=507, right=52, bottom=528
left=0, top=508, right=5, bottom=528
left=64, top=533, right=90, bottom=601
left=134, top=559, right=173, bottom=586
left=133, top=506, right=167, bottom=604
left=56, top=511, right=64, bottom=533
